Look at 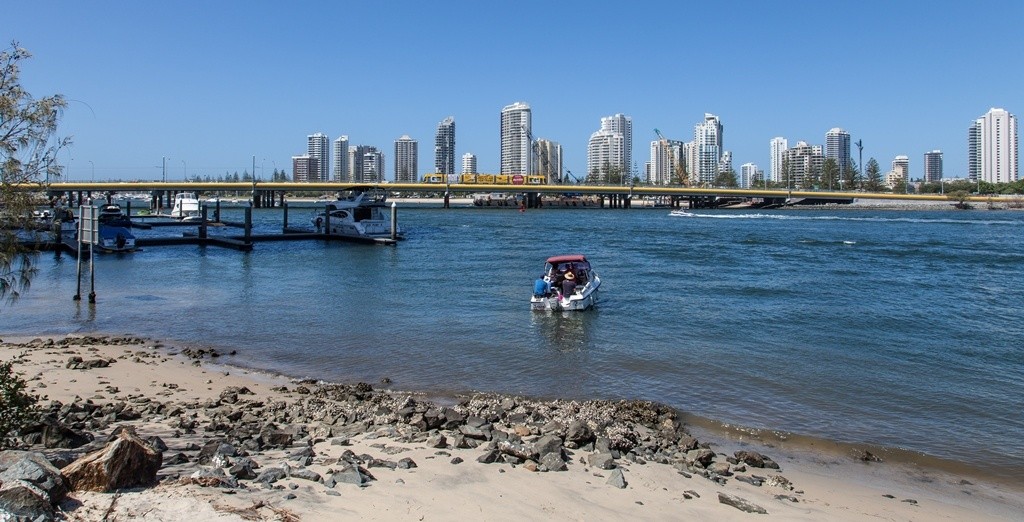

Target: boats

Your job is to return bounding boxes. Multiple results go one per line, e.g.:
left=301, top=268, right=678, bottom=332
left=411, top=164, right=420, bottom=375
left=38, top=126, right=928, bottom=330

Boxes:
left=170, top=191, right=203, bottom=219
left=91, top=191, right=153, bottom=209
left=312, top=191, right=401, bottom=239
left=73, top=202, right=136, bottom=250
left=530, top=256, right=601, bottom=312
left=200, top=199, right=252, bottom=208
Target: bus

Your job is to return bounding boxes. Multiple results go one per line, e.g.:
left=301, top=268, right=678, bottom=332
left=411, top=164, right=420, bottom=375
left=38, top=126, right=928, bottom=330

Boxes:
left=423, top=174, right=546, bottom=184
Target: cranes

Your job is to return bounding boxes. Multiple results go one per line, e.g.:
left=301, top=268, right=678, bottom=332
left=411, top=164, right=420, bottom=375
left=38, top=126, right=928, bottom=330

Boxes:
left=520, top=124, right=580, bottom=184
left=654, top=128, right=693, bottom=186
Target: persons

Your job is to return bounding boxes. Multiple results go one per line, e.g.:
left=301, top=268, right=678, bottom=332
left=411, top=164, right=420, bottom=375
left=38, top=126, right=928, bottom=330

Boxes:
left=473, top=195, right=527, bottom=206
left=536, top=194, right=543, bottom=208
left=533, top=264, right=579, bottom=300
left=60, top=193, right=68, bottom=206
left=698, top=198, right=720, bottom=209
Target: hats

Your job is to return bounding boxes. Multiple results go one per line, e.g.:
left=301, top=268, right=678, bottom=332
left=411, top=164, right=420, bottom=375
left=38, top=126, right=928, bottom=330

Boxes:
left=544, top=276, right=551, bottom=282
left=564, top=271, right=574, bottom=280
